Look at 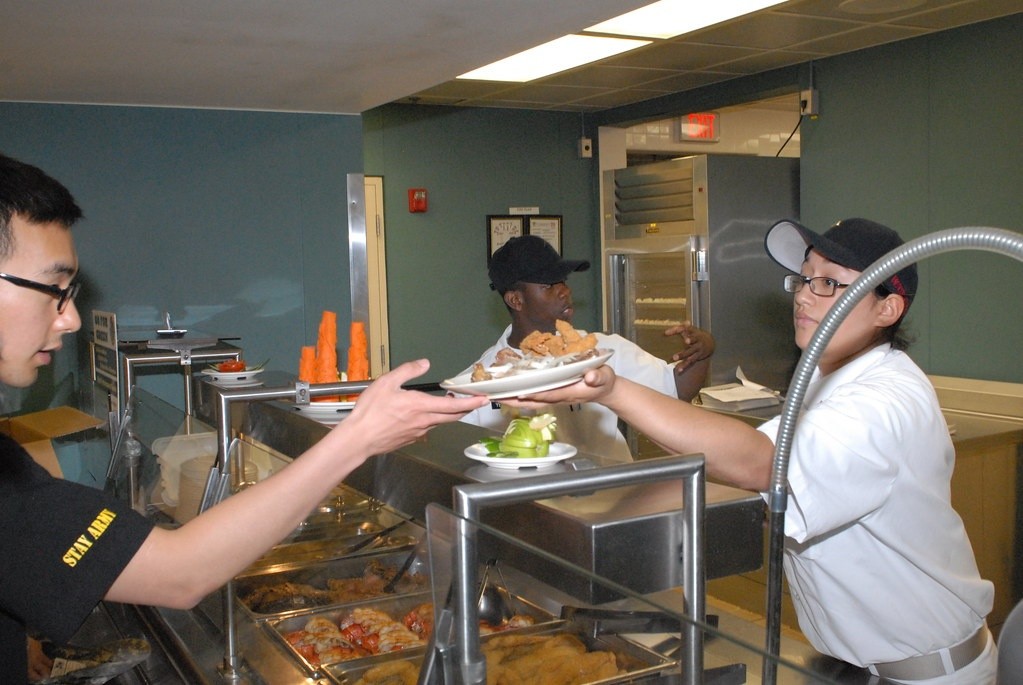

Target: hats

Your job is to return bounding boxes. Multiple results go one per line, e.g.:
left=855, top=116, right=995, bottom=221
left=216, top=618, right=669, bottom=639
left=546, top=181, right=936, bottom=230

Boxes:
left=765, top=218, right=917, bottom=316
left=488, top=235, right=589, bottom=292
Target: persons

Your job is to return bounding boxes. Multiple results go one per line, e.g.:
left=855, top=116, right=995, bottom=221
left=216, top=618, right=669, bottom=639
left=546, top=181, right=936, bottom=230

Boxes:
left=0, top=154, right=489, bottom=685
left=495, top=218, right=997, bottom=685
left=447, top=235, right=715, bottom=464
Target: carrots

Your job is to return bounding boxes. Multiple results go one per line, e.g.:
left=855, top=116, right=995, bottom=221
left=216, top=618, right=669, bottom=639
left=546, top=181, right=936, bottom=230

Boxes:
left=298, top=312, right=369, bottom=402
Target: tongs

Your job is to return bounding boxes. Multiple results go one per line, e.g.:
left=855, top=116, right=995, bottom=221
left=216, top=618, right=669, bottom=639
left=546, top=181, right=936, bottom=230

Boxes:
left=478, top=557, right=515, bottom=617
left=559, top=605, right=720, bottom=640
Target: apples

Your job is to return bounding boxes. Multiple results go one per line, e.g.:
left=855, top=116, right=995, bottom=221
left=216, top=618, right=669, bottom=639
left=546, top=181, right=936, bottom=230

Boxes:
left=498, top=419, right=548, bottom=458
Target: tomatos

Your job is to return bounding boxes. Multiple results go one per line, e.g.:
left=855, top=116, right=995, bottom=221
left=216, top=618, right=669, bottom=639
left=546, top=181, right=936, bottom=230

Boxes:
left=217, top=360, right=243, bottom=372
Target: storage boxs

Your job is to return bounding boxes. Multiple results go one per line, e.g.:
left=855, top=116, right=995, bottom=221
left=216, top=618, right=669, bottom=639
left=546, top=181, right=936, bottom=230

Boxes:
left=0, top=405, right=107, bottom=480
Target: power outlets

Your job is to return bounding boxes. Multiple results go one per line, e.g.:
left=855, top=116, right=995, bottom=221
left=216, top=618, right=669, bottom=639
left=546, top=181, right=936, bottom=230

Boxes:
left=800, top=89, right=819, bottom=116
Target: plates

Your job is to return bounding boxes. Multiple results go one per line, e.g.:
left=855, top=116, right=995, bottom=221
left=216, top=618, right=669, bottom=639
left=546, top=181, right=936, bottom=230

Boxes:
left=463, top=441, right=578, bottom=469
left=201, top=365, right=264, bottom=380
left=295, top=402, right=356, bottom=412
left=202, top=379, right=265, bottom=388
left=439, top=348, right=615, bottom=399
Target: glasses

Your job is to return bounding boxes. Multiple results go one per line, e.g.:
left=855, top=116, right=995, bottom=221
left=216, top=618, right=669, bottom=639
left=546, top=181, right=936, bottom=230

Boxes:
left=783, top=274, right=851, bottom=297
left=0, top=273, right=79, bottom=312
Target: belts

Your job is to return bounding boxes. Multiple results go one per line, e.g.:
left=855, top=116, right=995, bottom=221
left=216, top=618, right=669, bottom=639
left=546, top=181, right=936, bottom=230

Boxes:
left=863, top=622, right=988, bottom=680
